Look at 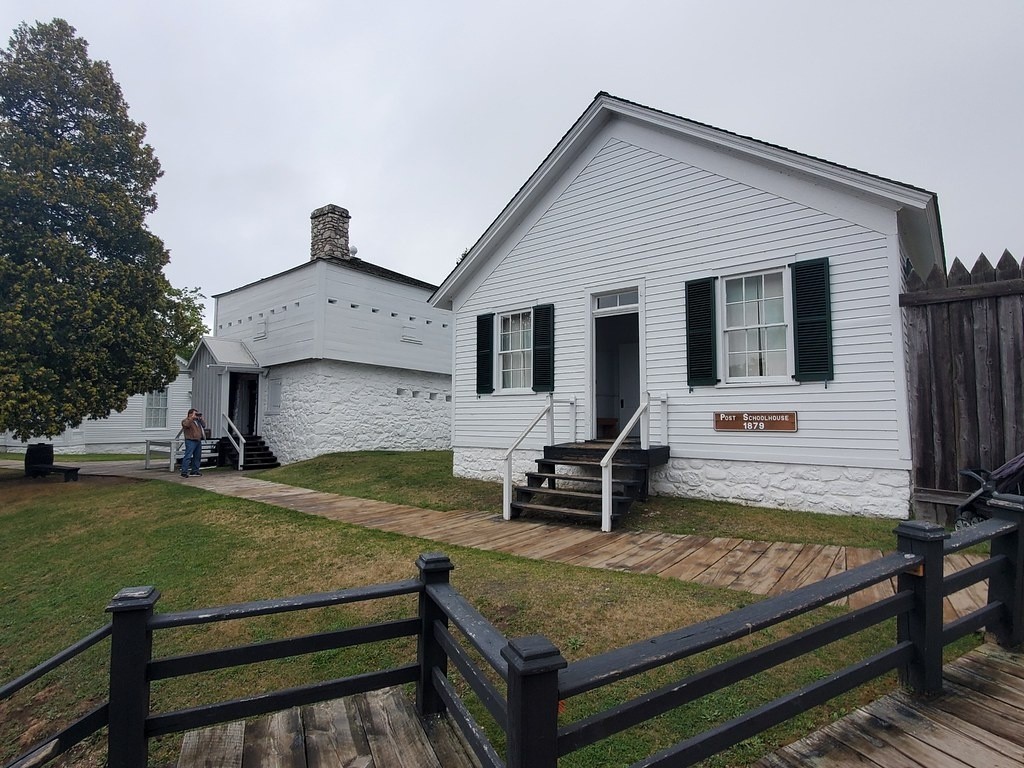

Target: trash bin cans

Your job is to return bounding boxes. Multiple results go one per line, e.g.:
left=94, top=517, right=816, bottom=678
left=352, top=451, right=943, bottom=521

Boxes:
left=24, top=443, right=54, bottom=476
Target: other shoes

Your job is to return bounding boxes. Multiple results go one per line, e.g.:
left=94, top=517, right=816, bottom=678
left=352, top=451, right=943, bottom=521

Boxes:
left=189, top=472, right=202, bottom=476
left=180, top=473, right=189, bottom=479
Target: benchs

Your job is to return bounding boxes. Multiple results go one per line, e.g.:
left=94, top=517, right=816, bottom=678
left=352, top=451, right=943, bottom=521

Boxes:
left=33, top=464, right=80, bottom=482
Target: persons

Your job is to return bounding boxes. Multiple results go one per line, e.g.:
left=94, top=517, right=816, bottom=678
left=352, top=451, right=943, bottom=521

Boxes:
left=181, top=409, right=205, bottom=479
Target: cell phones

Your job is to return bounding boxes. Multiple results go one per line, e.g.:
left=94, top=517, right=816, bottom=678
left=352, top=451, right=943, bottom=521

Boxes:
left=196, top=412, right=202, bottom=418
